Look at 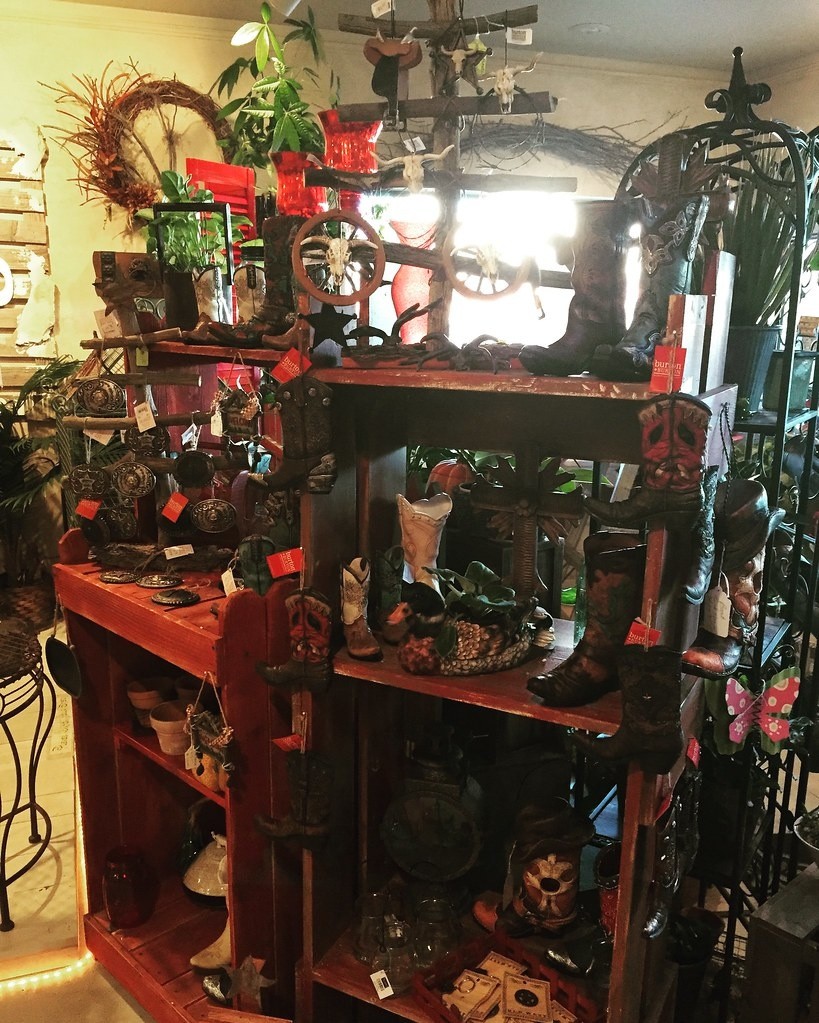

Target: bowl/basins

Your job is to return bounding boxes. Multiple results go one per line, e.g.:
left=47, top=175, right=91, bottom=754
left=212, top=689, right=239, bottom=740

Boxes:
left=127, top=675, right=174, bottom=728
left=175, top=677, right=212, bottom=703
left=148, top=700, right=205, bottom=755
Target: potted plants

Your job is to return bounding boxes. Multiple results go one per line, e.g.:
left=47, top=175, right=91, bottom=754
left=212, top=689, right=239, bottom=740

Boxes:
left=791, top=812, right=819, bottom=871
left=698, top=667, right=764, bottom=858
left=134, top=170, right=254, bottom=329
left=208, top=2, right=328, bottom=218
left=306, top=66, right=383, bottom=175
left=689, top=132, right=797, bottom=419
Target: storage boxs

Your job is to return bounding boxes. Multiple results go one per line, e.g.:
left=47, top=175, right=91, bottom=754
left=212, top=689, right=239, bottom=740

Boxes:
left=667, top=293, right=708, bottom=396
left=699, top=250, right=737, bottom=392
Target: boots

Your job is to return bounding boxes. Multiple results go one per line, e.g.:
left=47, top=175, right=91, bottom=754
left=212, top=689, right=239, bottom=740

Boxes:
left=190, top=855, right=234, bottom=1002
left=257, top=586, right=333, bottom=694
left=677, top=464, right=717, bottom=606
left=183, top=216, right=307, bottom=348
left=526, top=532, right=646, bottom=706
left=396, top=492, right=452, bottom=623
left=472, top=773, right=726, bottom=1020
left=519, top=199, right=628, bottom=374
left=570, top=644, right=684, bottom=774
left=582, top=393, right=713, bottom=535
left=246, top=375, right=340, bottom=495
left=683, top=546, right=765, bottom=680
left=370, top=546, right=409, bottom=644
left=254, top=748, right=336, bottom=836
left=341, top=556, right=380, bottom=658
left=590, top=203, right=700, bottom=378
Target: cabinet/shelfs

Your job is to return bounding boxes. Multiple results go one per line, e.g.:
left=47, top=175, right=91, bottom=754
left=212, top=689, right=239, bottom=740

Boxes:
left=303, top=0, right=578, bottom=351
left=49, top=338, right=736, bottom=1023
left=608, top=127, right=819, bottom=1023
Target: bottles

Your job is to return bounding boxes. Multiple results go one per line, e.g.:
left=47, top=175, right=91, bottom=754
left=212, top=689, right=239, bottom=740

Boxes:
left=101, top=844, right=153, bottom=929
left=349, top=892, right=457, bottom=996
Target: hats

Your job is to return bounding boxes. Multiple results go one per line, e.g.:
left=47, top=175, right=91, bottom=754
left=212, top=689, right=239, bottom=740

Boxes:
left=714, top=479, right=785, bottom=573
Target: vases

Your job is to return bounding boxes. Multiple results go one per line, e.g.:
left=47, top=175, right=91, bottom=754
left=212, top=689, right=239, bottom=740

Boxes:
left=762, top=350, right=819, bottom=412
